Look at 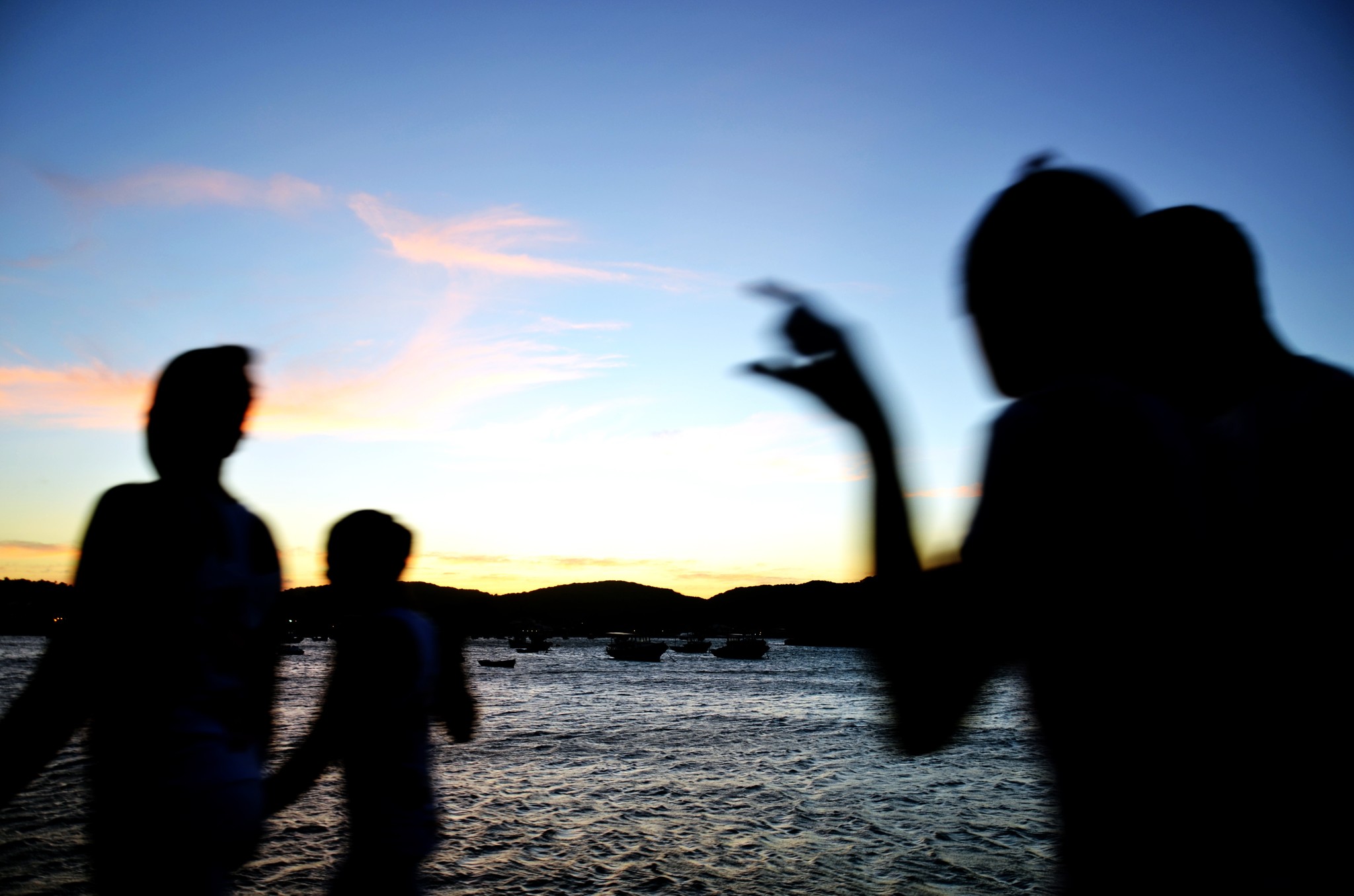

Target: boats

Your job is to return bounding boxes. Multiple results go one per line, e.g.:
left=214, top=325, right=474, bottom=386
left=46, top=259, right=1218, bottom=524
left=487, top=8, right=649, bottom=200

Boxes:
left=516, top=648, right=539, bottom=653
left=282, top=636, right=305, bottom=644
left=477, top=658, right=516, bottom=668
left=669, top=634, right=712, bottom=654
left=311, top=637, right=328, bottom=641
left=508, top=638, right=553, bottom=651
left=604, top=632, right=669, bottom=663
left=281, top=645, right=304, bottom=655
left=710, top=634, right=771, bottom=660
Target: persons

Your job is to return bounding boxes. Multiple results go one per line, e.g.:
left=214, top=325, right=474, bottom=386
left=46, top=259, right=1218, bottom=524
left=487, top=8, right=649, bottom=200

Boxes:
left=734, top=147, right=1354, bottom=896
left=263, top=508, right=480, bottom=896
left=0, top=345, right=285, bottom=896
